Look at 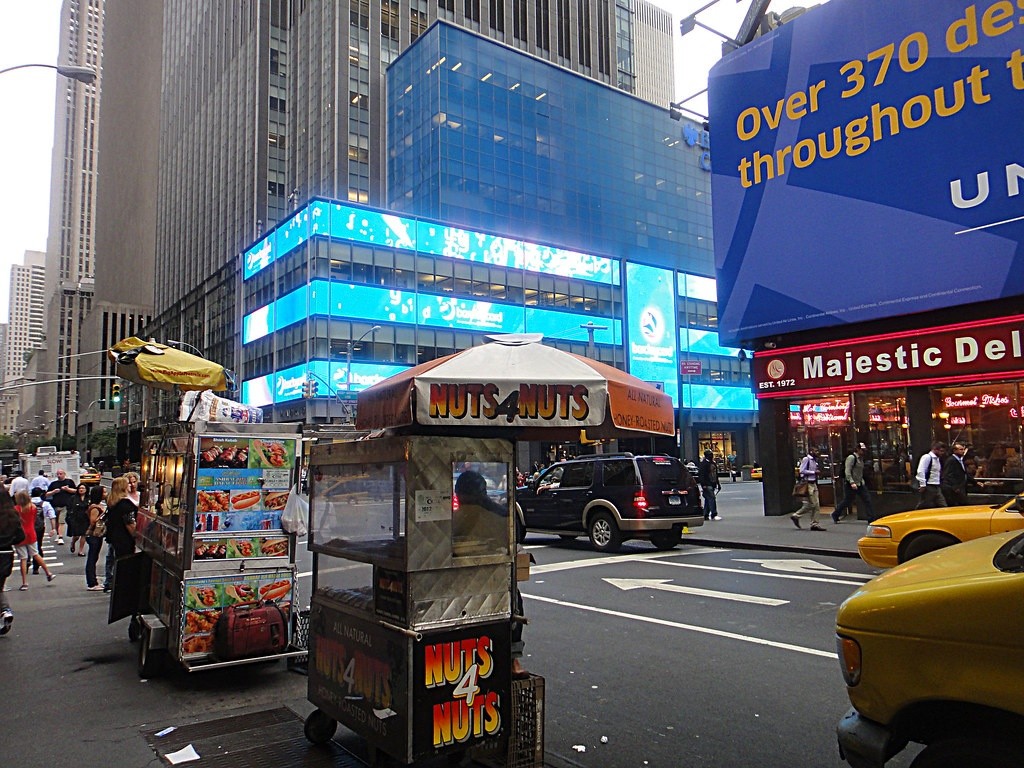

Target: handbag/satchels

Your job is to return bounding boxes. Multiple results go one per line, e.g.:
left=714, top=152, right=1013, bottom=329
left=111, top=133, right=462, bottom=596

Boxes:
left=792, top=481, right=808, bottom=497
left=912, top=478, right=924, bottom=494
left=93, top=512, right=108, bottom=539
left=280, top=483, right=309, bottom=536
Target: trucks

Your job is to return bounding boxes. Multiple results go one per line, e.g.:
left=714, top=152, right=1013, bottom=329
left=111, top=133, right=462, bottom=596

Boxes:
left=19, top=446, right=81, bottom=489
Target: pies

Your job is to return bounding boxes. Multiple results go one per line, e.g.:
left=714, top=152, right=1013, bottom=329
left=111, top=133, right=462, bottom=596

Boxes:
left=254, top=441, right=289, bottom=467
left=262, top=539, right=288, bottom=556
left=190, top=586, right=217, bottom=607
left=226, top=584, right=255, bottom=602
left=265, top=492, right=290, bottom=510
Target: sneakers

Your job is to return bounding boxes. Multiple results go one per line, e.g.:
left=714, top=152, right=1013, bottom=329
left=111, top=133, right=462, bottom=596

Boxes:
left=0, top=611, right=14, bottom=635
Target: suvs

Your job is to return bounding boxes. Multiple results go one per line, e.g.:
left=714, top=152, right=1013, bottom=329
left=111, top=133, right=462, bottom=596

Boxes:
left=498, top=452, right=706, bottom=553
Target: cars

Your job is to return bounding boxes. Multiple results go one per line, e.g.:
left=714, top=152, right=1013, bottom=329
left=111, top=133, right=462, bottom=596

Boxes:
left=750, top=467, right=762, bottom=482
left=81, top=462, right=101, bottom=487
left=836, top=529, right=1024, bottom=768
left=129, top=462, right=141, bottom=471
left=857, top=492, right=1024, bottom=568
left=451, top=471, right=507, bottom=515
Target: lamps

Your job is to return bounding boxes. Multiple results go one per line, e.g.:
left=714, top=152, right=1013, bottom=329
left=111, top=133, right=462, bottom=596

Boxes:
left=670, top=89, right=709, bottom=133
left=680, top=0, right=745, bottom=48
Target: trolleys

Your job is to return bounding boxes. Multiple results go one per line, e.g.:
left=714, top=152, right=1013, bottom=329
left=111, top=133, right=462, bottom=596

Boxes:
left=105, top=421, right=307, bottom=682
left=303, top=435, right=527, bottom=767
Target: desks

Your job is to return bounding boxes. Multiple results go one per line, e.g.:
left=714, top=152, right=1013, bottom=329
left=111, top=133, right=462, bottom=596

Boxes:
left=885, top=481, right=911, bottom=490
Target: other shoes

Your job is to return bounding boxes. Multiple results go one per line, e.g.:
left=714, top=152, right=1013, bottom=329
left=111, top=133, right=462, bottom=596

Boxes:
left=831, top=512, right=838, bottom=524
left=712, top=515, right=722, bottom=521
left=49, top=530, right=85, bottom=557
left=811, top=525, right=826, bottom=531
left=88, top=584, right=104, bottom=591
left=791, top=515, right=801, bottom=529
left=2, top=563, right=56, bottom=591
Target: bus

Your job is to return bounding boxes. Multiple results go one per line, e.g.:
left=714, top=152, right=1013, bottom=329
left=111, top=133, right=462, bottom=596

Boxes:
left=0, top=449, right=19, bottom=479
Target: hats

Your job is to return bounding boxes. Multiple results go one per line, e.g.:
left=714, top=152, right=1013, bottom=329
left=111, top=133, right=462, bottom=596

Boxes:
left=32, top=488, right=43, bottom=496
left=857, top=442, right=869, bottom=451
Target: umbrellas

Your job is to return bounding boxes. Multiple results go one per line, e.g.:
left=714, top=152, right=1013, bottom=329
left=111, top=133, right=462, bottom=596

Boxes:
left=107, top=337, right=235, bottom=428
left=355, top=332, right=675, bottom=551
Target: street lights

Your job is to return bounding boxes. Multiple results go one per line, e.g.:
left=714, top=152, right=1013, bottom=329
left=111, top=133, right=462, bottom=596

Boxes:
left=11, top=410, right=78, bottom=454
left=65, top=398, right=106, bottom=462
left=346, top=325, right=382, bottom=424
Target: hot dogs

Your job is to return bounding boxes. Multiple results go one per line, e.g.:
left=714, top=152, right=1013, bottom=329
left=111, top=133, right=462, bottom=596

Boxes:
left=231, top=491, right=261, bottom=509
left=230, top=540, right=255, bottom=556
left=259, top=580, right=292, bottom=600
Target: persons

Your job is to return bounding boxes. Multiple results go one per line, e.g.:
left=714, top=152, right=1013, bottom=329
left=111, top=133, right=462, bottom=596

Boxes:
left=515, top=460, right=545, bottom=488
left=536, top=471, right=562, bottom=494
left=454, top=470, right=509, bottom=517
left=830, top=443, right=875, bottom=525
left=697, top=449, right=722, bottom=520
left=915, top=442, right=947, bottom=510
left=0, top=468, right=141, bottom=635
left=790, top=447, right=827, bottom=531
left=940, top=444, right=983, bottom=507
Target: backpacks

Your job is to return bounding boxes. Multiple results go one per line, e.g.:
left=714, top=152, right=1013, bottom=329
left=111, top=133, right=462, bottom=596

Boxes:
left=79, top=506, right=101, bottom=534
left=33, top=500, right=44, bottom=531
left=839, top=454, right=857, bottom=479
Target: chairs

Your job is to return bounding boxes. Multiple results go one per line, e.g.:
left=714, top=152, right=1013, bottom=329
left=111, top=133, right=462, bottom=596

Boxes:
left=871, top=471, right=886, bottom=492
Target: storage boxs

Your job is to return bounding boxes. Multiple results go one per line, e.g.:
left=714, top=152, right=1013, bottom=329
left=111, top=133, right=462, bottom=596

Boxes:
left=470, top=671, right=545, bottom=768
left=287, top=606, right=310, bottom=674
left=517, top=552, right=537, bottom=582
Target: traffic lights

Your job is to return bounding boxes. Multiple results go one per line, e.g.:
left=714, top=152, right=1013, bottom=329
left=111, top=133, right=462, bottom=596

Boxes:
left=311, top=380, right=319, bottom=400
left=302, top=382, right=310, bottom=399
left=113, top=384, right=120, bottom=402
